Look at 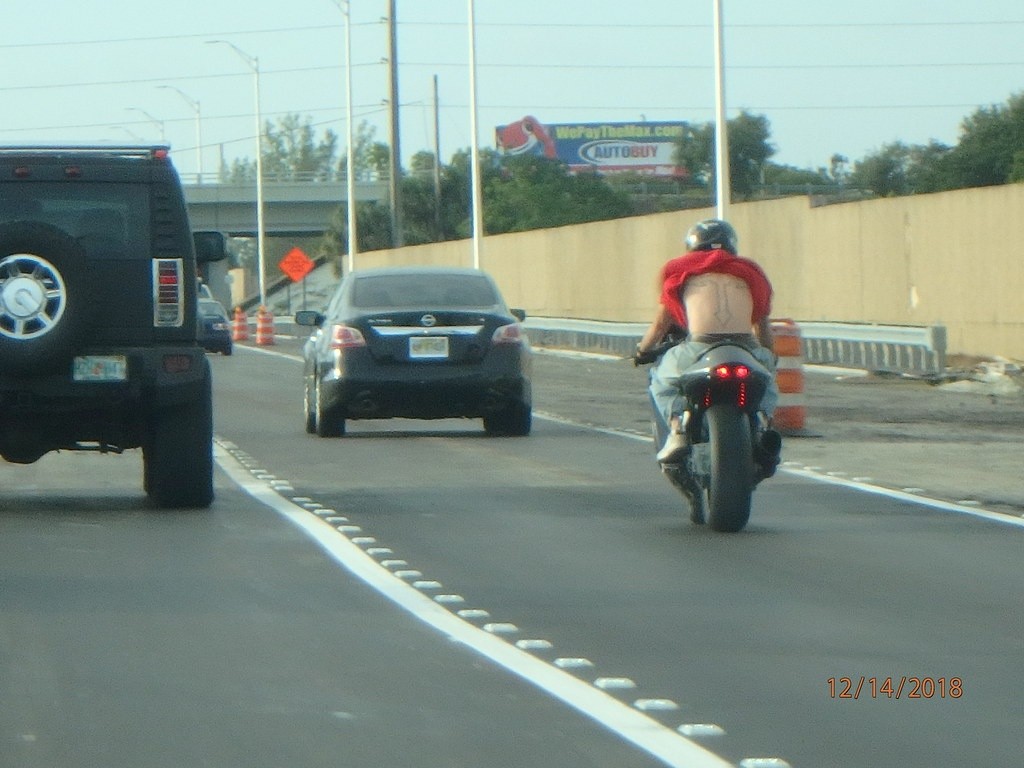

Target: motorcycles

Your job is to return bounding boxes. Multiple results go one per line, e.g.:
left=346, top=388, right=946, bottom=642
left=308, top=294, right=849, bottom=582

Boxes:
left=633, top=333, right=786, bottom=532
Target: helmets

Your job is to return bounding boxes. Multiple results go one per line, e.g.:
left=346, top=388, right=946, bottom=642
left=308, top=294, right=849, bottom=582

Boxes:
left=686, top=219, right=739, bottom=256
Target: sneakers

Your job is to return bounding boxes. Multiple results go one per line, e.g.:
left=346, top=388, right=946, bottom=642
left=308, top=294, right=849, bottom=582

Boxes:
left=655, top=429, right=691, bottom=464
left=756, top=430, right=782, bottom=479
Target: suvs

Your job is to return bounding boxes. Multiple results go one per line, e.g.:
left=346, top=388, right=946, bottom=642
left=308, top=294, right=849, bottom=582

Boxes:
left=0, top=138, right=230, bottom=511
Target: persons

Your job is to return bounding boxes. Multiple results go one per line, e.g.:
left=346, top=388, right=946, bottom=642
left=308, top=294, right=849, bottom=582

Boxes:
left=636, top=218, right=778, bottom=463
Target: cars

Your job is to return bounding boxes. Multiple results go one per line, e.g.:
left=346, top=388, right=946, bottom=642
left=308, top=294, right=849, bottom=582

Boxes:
left=289, top=265, right=536, bottom=439
left=195, top=297, right=233, bottom=357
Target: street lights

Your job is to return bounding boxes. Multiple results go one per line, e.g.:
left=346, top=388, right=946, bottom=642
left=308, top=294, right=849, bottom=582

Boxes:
left=209, top=38, right=268, bottom=308
left=127, top=106, right=166, bottom=142
left=159, top=85, right=203, bottom=179
